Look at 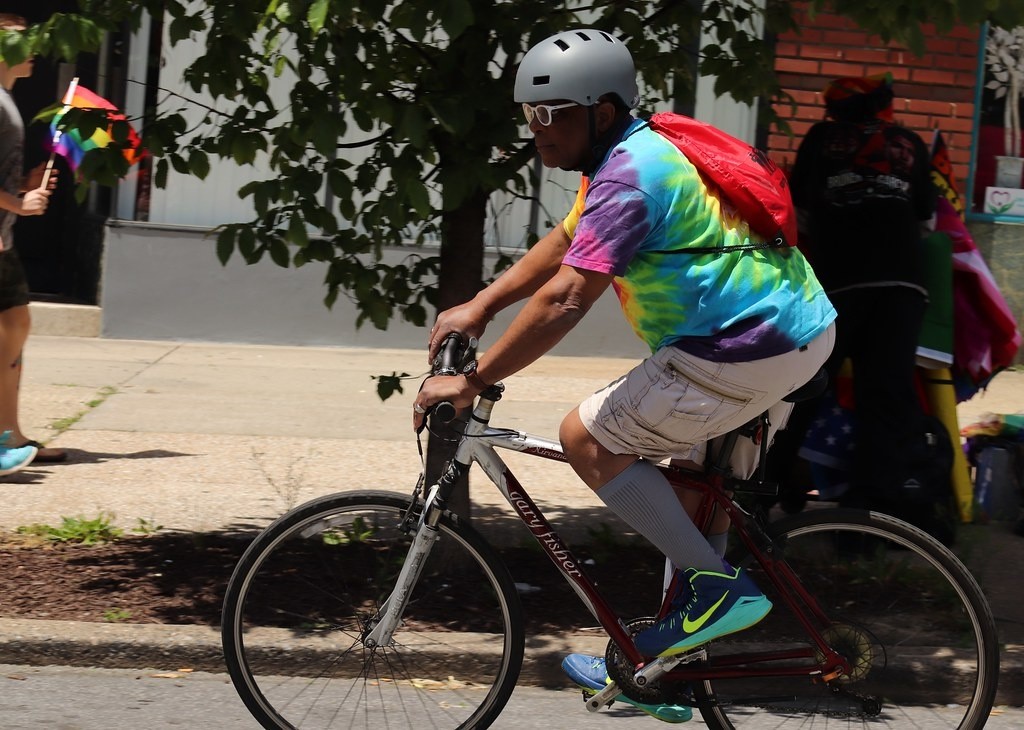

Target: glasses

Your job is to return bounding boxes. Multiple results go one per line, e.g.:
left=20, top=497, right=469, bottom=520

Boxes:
left=522, top=100, right=600, bottom=125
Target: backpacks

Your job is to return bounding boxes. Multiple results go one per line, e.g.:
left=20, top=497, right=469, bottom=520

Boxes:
left=623, top=112, right=797, bottom=256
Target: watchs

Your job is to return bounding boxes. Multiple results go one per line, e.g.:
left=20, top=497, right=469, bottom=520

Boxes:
left=463, top=359, right=491, bottom=390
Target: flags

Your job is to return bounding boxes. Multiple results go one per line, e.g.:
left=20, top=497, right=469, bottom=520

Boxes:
left=48, top=82, right=151, bottom=185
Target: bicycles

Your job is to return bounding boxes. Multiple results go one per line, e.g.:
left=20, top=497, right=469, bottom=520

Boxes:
left=221, top=330, right=1001, bottom=730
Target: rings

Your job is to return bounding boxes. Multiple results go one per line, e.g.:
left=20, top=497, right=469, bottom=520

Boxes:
left=415, top=403, right=425, bottom=414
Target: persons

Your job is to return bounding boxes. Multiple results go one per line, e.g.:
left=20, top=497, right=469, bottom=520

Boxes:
left=914, top=232, right=978, bottom=523
left=775, top=77, right=962, bottom=552
left=883, top=124, right=928, bottom=177
left=413, top=29, right=838, bottom=723
left=0, top=14, right=70, bottom=462
left=0, top=430, right=38, bottom=476
left=834, top=134, right=875, bottom=170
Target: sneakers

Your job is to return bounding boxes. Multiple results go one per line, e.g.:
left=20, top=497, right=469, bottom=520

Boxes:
left=0, top=442, right=38, bottom=476
left=563, top=653, right=692, bottom=723
left=636, top=570, right=773, bottom=658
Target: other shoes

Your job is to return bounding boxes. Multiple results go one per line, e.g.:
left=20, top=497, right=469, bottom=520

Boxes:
left=16, top=440, right=69, bottom=462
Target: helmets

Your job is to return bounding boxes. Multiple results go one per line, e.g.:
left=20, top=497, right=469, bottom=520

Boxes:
left=514, top=29, right=642, bottom=109
